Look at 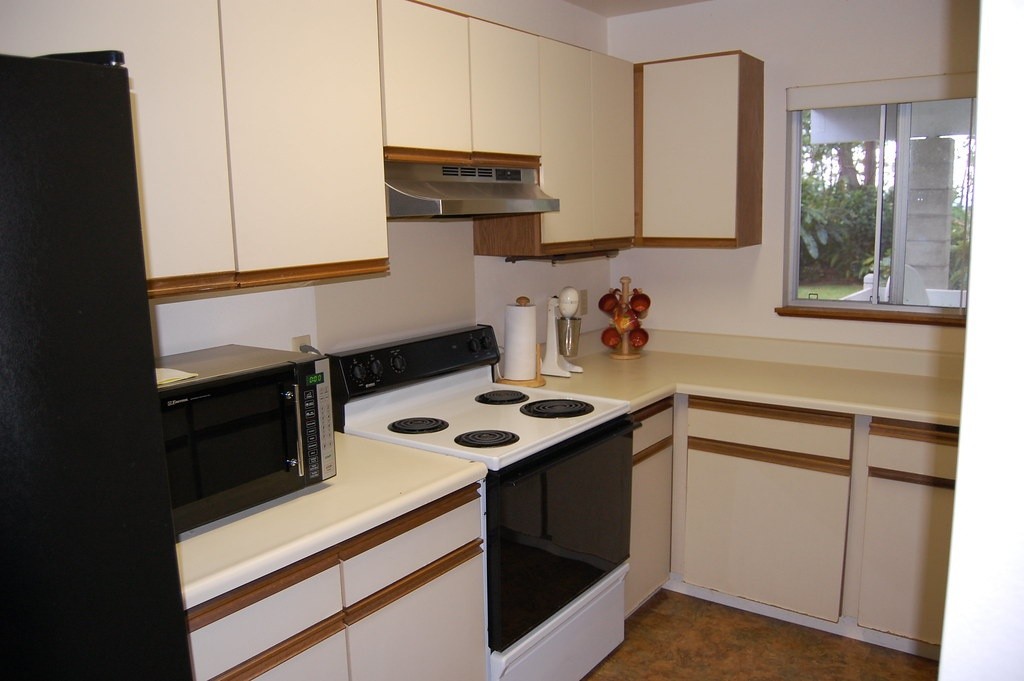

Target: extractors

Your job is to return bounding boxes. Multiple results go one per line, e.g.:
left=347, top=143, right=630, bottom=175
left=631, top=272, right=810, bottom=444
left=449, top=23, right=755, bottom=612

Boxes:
left=384, top=160, right=560, bottom=222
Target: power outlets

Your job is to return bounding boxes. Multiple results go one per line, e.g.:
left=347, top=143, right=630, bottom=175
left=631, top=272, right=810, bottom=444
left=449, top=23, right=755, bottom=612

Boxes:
left=578, top=289, right=588, bottom=317
left=291, top=334, right=310, bottom=354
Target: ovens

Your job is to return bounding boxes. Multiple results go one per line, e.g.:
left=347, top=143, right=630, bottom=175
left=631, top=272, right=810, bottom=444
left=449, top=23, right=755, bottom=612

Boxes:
left=484, top=415, right=642, bottom=681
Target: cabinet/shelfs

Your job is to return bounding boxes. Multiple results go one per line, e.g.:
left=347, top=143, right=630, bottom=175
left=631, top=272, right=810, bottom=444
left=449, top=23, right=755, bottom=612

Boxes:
left=636, top=49, right=765, bottom=249
left=677, top=392, right=856, bottom=639
left=0, top=0, right=391, bottom=298
left=183, top=481, right=488, bottom=681
left=629, top=395, right=681, bottom=621
left=857, top=416, right=960, bottom=661
left=473, top=36, right=637, bottom=256
left=376, top=0, right=542, bottom=168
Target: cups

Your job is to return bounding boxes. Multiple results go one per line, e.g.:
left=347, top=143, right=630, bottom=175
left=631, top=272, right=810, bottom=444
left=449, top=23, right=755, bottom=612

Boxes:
left=557, top=317, right=581, bottom=357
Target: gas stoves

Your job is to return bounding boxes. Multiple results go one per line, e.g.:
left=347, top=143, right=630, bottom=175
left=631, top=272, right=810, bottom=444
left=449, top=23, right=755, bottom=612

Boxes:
left=324, top=325, right=627, bottom=472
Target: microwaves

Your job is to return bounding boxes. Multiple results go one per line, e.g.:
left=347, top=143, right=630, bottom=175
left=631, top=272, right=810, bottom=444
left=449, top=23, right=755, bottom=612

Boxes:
left=154, top=344, right=337, bottom=535
